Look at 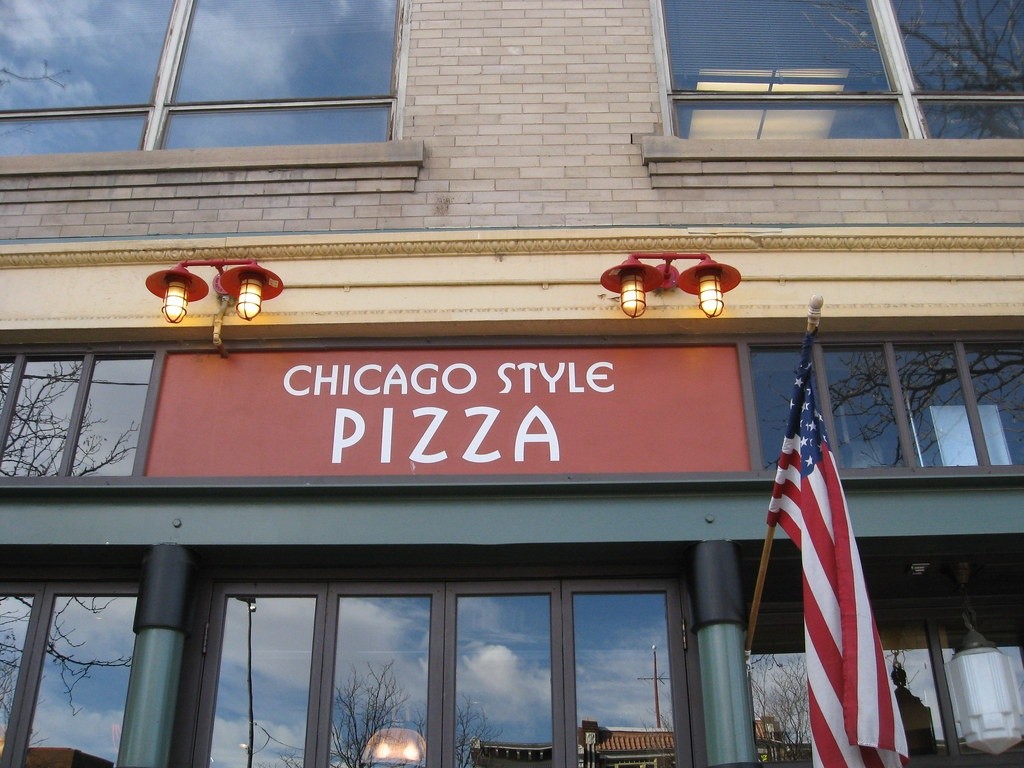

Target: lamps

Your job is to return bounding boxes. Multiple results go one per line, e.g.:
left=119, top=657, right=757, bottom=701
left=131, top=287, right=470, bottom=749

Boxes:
left=601, top=253, right=741, bottom=319
left=940, top=558, right=1024, bottom=756
left=358, top=704, right=426, bottom=765
left=889, top=649, right=937, bottom=755
left=144, top=259, right=284, bottom=326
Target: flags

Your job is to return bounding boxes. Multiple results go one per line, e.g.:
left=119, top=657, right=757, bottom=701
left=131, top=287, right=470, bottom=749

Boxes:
left=766, top=330, right=911, bottom=768
